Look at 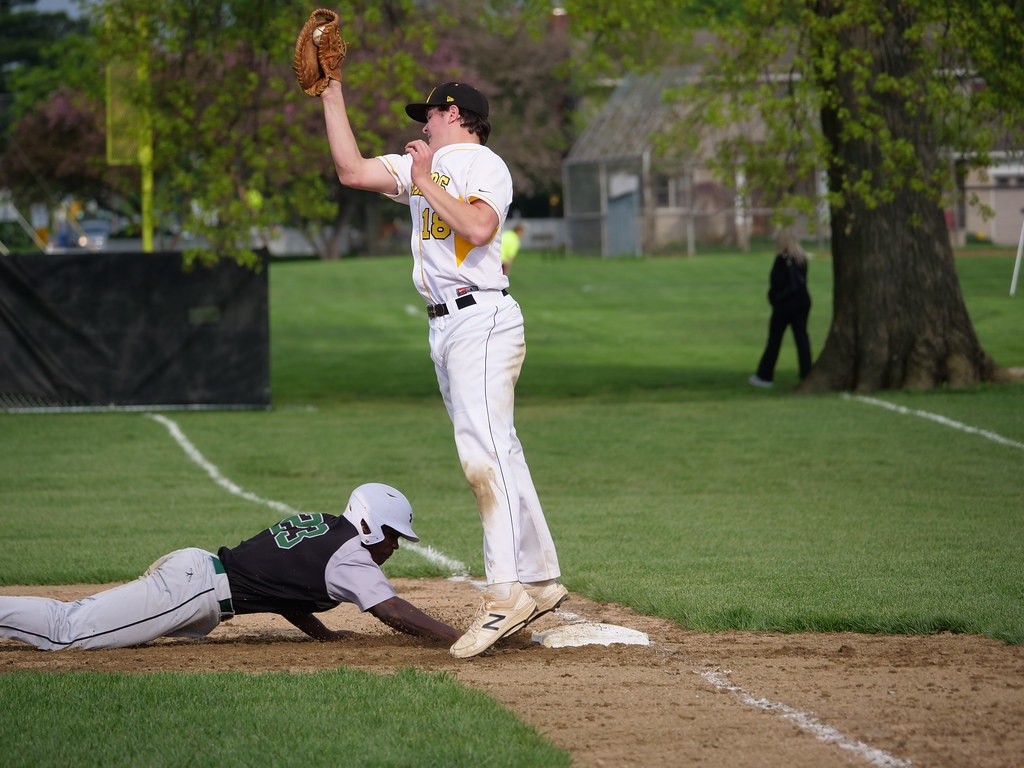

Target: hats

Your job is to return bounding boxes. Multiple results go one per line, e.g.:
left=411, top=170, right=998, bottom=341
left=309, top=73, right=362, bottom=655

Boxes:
left=406, top=83, right=489, bottom=124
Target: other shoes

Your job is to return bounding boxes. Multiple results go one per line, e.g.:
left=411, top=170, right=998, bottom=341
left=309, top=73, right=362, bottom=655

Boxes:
left=750, top=377, right=773, bottom=387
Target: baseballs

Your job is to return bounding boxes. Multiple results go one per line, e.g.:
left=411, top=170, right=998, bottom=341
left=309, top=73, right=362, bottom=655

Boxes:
left=312, top=24, right=325, bottom=46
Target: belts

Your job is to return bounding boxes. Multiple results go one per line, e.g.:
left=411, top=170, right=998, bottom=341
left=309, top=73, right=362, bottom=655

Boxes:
left=427, top=289, right=508, bottom=318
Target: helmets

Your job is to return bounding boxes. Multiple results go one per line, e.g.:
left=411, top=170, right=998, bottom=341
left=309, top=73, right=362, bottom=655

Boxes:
left=342, top=483, right=420, bottom=545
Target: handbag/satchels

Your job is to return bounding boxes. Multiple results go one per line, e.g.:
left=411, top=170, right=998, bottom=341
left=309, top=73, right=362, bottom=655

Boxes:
left=782, top=254, right=805, bottom=296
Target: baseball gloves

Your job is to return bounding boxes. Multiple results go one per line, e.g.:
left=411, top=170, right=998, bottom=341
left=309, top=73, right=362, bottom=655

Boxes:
left=294, top=8, right=349, bottom=97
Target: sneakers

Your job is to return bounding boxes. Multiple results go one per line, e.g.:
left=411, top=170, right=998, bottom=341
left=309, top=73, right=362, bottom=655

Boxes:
left=499, top=579, right=568, bottom=638
left=450, top=582, right=538, bottom=658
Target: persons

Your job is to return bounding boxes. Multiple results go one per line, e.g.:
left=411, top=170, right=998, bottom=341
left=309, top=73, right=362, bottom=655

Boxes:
left=0, top=483, right=534, bottom=651
left=501, top=225, right=524, bottom=275
left=750, top=227, right=813, bottom=388
left=294, top=6, right=567, bottom=661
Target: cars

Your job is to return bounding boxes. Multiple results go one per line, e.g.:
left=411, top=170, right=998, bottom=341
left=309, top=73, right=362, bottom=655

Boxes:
left=103, top=216, right=213, bottom=250
left=48, top=221, right=109, bottom=253
